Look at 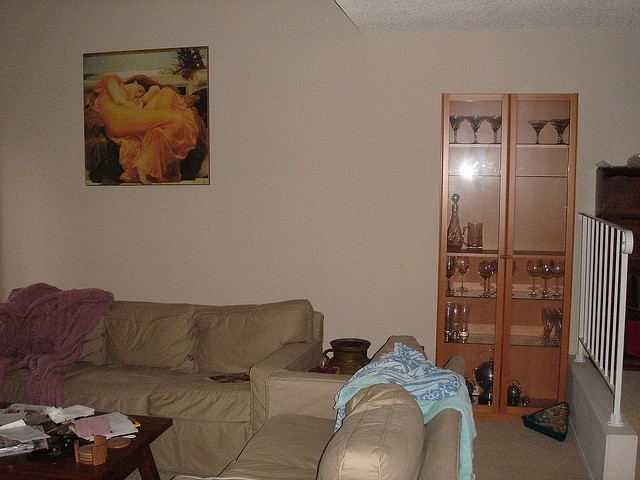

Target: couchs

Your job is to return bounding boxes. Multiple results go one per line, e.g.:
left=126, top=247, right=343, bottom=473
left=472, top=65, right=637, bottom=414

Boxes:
left=220, top=335, right=464, bottom=480
left=0, top=288, right=323, bottom=478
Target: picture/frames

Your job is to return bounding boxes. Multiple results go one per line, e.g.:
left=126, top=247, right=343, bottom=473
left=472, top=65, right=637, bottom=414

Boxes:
left=84, top=45, right=213, bottom=186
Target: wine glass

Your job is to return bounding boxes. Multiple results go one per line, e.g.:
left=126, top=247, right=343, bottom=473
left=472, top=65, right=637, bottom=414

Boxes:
left=478, top=259, right=498, bottom=298
left=485, top=115, right=502, bottom=144
left=456, top=257, right=469, bottom=295
left=450, top=114, right=466, bottom=143
left=540, top=307, right=562, bottom=345
left=446, top=256, right=455, bottom=297
left=528, top=120, right=549, bottom=143
left=466, top=114, right=485, bottom=143
left=444, top=301, right=470, bottom=343
left=549, top=119, right=570, bottom=144
left=526, top=258, right=564, bottom=299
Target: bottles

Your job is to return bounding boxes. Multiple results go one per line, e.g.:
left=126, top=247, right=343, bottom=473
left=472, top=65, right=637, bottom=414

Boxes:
left=447, top=194, right=463, bottom=253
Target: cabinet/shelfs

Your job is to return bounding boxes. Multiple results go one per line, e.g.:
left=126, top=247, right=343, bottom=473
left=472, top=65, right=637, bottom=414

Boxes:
left=589, top=166, right=639, bottom=371
left=435, top=93, right=578, bottom=423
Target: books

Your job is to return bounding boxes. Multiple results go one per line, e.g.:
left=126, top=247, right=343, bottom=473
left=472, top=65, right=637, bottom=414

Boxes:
left=69, top=411, right=141, bottom=438
left=0, top=425, right=48, bottom=458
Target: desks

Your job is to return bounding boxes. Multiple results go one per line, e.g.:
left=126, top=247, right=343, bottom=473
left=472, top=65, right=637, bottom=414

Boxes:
left=0, top=403, right=174, bottom=480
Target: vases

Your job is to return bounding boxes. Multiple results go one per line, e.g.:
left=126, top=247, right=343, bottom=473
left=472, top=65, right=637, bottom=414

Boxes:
left=321, top=338, right=371, bottom=375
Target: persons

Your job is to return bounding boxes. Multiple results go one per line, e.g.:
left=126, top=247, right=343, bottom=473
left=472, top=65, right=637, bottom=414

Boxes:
left=88, top=74, right=200, bottom=184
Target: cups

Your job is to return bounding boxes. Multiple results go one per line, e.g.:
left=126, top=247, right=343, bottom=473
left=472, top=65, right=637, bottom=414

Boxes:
left=462, top=222, right=485, bottom=250
